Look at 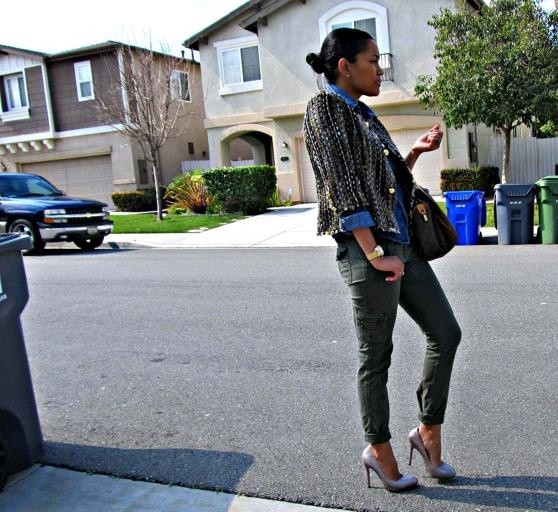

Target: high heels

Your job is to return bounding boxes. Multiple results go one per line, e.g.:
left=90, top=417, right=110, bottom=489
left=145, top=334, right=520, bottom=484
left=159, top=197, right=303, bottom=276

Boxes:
left=362, top=446, right=418, bottom=492
left=408, top=427, right=456, bottom=479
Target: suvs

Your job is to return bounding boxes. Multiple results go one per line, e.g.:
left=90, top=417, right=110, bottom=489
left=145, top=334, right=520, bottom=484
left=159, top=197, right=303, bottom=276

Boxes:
left=0, top=170, right=111, bottom=251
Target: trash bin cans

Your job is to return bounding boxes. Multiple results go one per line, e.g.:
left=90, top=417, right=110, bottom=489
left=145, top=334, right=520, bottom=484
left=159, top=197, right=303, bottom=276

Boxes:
left=534, top=176, right=558, bottom=244
left=442, top=190, right=486, bottom=245
left=0, top=231, right=43, bottom=492
left=494, top=184, right=540, bottom=245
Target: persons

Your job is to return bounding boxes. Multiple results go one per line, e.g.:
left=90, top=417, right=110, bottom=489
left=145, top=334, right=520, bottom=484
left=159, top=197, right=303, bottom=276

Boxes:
left=302, top=28, right=462, bottom=493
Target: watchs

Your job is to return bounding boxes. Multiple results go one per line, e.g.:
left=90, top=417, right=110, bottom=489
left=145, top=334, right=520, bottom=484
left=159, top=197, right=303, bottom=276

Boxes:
left=365, top=245, right=385, bottom=261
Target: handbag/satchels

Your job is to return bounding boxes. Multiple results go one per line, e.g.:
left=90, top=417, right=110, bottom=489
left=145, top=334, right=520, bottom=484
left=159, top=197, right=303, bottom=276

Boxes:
left=408, top=184, right=458, bottom=262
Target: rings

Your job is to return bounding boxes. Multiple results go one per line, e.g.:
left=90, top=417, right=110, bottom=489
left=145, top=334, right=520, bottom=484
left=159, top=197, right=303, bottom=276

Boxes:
left=399, top=271, right=405, bottom=275
left=436, top=137, right=442, bottom=146
